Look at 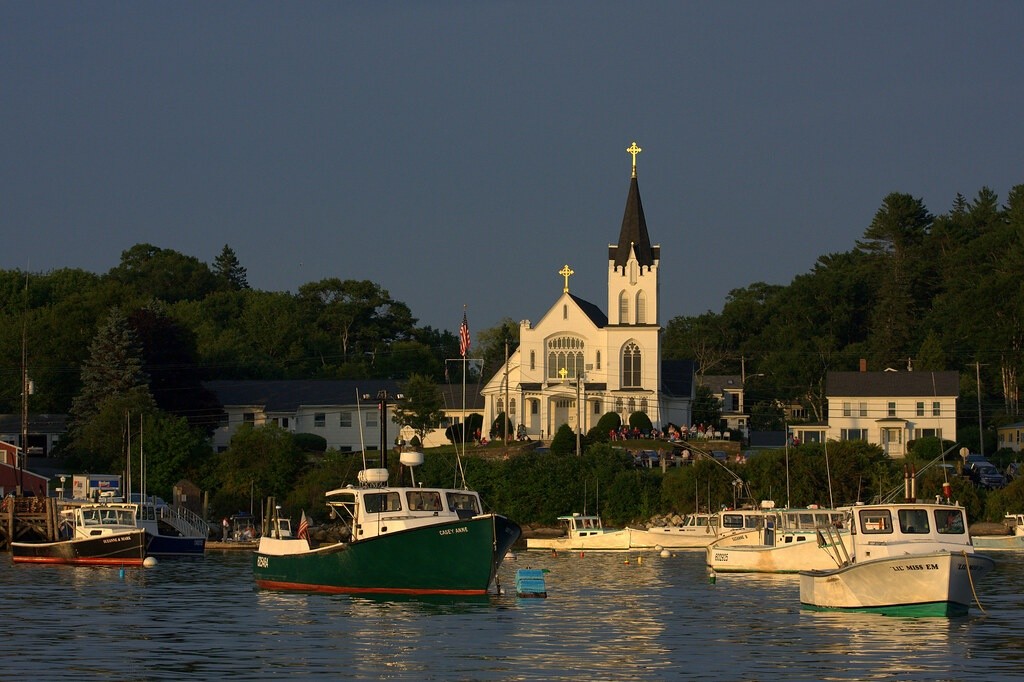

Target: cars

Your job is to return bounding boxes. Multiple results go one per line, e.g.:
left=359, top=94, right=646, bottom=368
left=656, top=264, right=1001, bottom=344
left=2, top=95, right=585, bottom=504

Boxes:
left=936, top=464, right=957, bottom=478
left=741, top=450, right=754, bottom=464
left=635, top=451, right=661, bottom=467
left=713, top=451, right=731, bottom=464
left=670, top=441, right=695, bottom=466
left=27, top=445, right=44, bottom=456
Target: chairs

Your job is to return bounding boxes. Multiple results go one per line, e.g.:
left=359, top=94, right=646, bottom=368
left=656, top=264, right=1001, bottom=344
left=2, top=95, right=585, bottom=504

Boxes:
left=715, top=432, right=722, bottom=440
left=723, top=431, right=730, bottom=441
left=706, top=432, right=714, bottom=440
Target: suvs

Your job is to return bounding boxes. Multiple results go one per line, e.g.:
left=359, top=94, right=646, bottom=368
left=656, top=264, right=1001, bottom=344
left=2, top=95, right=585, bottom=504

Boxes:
left=963, top=454, right=1003, bottom=489
left=1006, top=464, right=1021, bottom=481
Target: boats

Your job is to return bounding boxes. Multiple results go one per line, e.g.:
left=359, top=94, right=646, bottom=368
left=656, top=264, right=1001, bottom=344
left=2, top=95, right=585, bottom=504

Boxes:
left=254, top=388, right=522, bottom=606
left=526, top=475, right=1024, bottom=619
left=10, top=411, right=208, bottom=567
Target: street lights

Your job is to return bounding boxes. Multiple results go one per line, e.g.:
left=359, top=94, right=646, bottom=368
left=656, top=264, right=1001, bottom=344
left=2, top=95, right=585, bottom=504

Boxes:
left=60, top=476, right=66, bottom=500
left=742, top=373, right=764, bottom=384
left=576, top=371, right=589, bottom=455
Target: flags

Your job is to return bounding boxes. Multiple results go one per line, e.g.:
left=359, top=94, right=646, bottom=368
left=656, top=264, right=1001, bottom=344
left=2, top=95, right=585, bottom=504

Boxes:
left=460, top=311, right=471, bottom=356
left=297, top=510, right=311, bottom=546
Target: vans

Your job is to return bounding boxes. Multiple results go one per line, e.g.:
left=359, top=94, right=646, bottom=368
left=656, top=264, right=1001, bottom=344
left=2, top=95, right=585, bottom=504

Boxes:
left=127, top=493, right=153, bottom=514
left=150, top=497, right=169, bottom=516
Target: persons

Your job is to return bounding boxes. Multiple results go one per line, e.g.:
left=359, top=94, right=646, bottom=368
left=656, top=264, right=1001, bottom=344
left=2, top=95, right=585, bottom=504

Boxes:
left=681, top=424, right=688, bottom=438
left=473, top=428, right=495, bottom=448
left=222, top=515, right=229, bottom=542
left=632, top=427, right=640, bottom=440
left=690, top=424, right=697, bottom=438
left=240, top=526, right=255, bottom=542
left=707, top=424, right=716, bottom=439
left=735, top=452, right=747, bottom=465
left=680, top=447, right=690, bottom=465
left=507, top=433, right=513, bottom=442
left=609, top=428, right=618, bottom=442
left=626, top=447, right=715, bottom=468
left=660, top=425, right=680, bottom=442
left=785, top=435, right=801, bottom=448
left=619, top=427, right=629, bottom=441
left=651, top=428, right=659, bottom=440
left=698, top=423, right=705, bottom=441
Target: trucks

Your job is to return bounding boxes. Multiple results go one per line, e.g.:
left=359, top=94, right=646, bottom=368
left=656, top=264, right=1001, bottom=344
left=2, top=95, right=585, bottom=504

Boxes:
left=73, top=474, right=122, bottom=504
